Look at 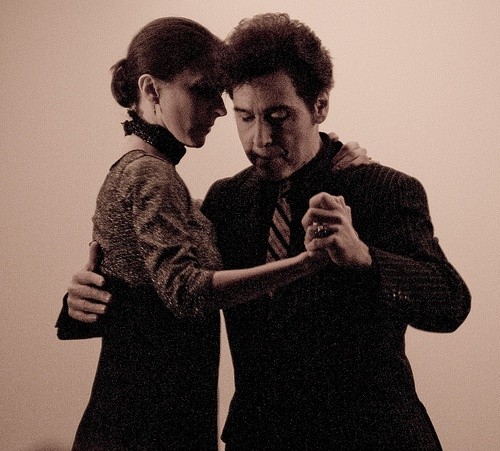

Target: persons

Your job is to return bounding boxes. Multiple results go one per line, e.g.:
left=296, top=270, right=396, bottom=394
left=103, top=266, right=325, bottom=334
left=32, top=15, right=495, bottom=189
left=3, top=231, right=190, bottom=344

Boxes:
left=70, top=17, right=377, bottom=451
left=52, top=11, right=473, bottom=451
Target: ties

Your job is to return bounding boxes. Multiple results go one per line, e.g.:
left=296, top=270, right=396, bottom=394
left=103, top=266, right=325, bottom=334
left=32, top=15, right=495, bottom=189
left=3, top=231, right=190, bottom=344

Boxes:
left=263, top=181, right=292, bottom=300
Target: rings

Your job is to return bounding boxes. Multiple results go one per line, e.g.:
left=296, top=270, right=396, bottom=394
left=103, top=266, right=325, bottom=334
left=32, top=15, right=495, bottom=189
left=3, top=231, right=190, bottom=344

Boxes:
left=368, top=157, right=372, bottom=161
left=314, top=223, right=330, bottom=238
